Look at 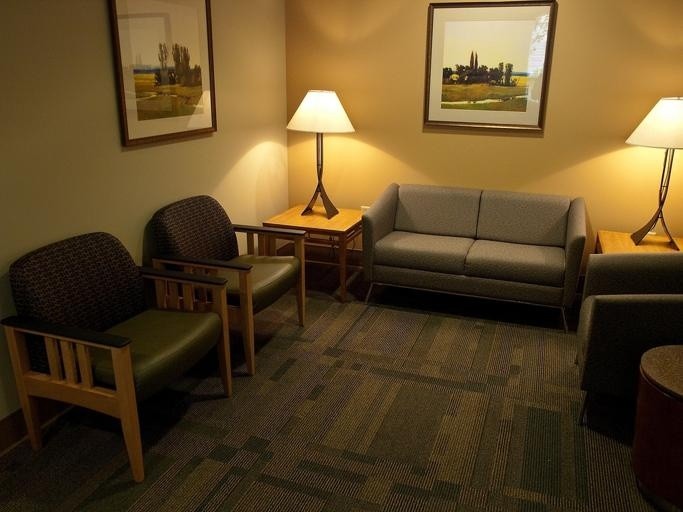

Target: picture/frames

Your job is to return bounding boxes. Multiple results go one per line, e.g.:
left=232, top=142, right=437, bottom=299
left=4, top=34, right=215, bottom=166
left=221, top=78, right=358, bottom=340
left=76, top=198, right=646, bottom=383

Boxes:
left=423, top=0, right=558, bottom=133
left=109, top=0, right=217, bottom=147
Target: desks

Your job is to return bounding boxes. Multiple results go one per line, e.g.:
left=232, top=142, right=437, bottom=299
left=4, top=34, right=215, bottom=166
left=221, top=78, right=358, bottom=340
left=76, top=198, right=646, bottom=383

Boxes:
left=632, top=344, right=683, bottom=512
left=263, top=205, right=362, bottom=303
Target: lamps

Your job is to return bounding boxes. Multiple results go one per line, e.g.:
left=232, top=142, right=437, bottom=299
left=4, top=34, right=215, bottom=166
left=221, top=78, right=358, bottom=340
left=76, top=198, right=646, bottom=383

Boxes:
left=286, top=89, right=356, bottom=220
left=625, top=95, right=683, bottom=250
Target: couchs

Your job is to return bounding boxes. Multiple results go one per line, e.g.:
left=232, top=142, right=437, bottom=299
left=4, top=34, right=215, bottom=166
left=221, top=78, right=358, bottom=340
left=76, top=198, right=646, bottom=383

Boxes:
left=363, top=182, right=588, bottom=334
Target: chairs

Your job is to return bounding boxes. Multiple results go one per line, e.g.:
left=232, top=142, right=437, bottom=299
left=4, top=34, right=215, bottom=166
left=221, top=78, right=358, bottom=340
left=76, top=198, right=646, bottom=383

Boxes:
left=575, top=252, right=682, bottom=429
left=147, top=195, right=309, bottom=376
left=2, top=231, right=233, bottom=484
